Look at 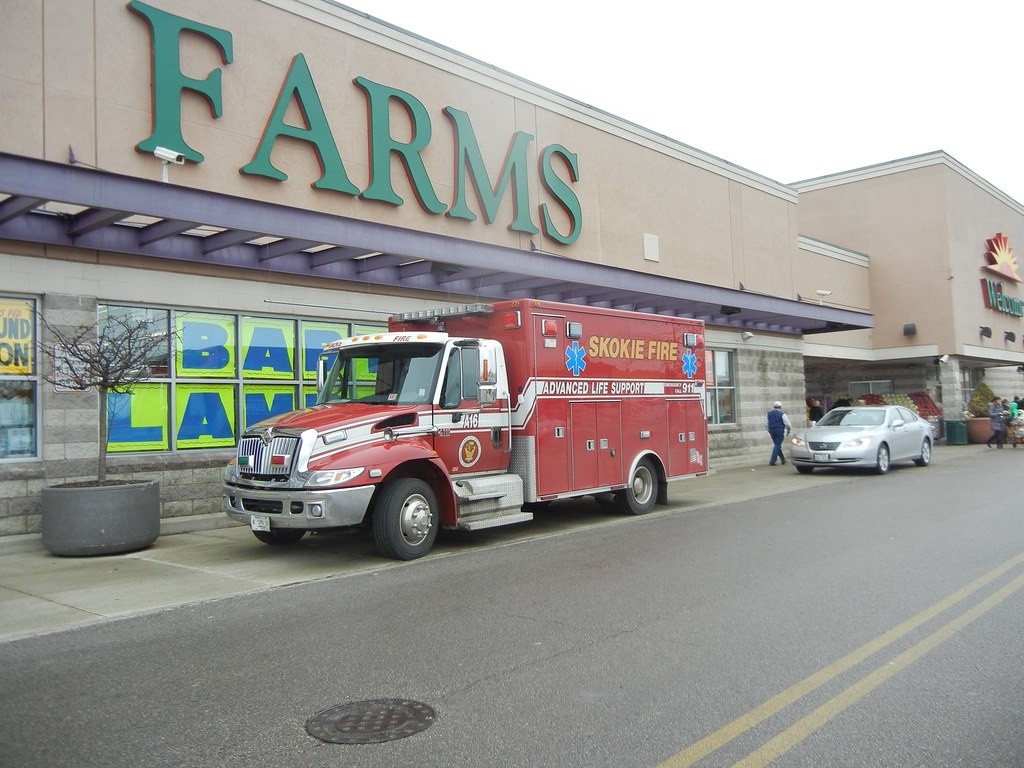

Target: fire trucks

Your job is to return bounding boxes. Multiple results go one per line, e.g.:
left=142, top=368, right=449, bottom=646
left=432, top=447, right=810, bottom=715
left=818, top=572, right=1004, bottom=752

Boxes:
left=223, top=297, right=709, bottom=562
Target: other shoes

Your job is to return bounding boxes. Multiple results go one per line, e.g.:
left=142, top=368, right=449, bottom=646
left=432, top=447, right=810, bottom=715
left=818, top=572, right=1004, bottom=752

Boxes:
left=770, top=463, right=778, bottom=466
left=985, top=440, right=992, bottom=448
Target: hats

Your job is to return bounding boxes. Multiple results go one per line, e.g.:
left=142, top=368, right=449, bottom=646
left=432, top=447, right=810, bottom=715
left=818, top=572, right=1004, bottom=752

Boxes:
left=773, top=400, right=783, bottom=407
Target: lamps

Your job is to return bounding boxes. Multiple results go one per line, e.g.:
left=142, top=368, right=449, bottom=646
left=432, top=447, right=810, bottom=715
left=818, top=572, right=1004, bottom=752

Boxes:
left=743, top=331, right=754, bottom=340
left=980, top=326, right=992, bottom=338
left=903, top=324, right=916, bottom=336
left=1005, top=331, right=1015, bottom=343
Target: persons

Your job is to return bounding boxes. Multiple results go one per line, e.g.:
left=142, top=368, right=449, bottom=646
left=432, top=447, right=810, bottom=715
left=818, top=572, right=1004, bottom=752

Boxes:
left=765, top=401, right=792, bottom=466
left=986, top=396, right=1024, bottom=449
left=809, top=400, right=824, bottom=424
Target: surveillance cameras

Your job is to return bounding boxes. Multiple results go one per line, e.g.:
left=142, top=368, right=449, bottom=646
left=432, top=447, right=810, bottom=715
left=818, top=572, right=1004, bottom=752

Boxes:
left=940, top=354, right=949, bottom=363
left=154, top=146, right=185, bottom=165
left=816, top=290, right=833, bottom=296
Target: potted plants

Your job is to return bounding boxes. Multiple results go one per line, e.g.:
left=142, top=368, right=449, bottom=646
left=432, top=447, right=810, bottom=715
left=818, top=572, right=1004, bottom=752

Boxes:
left=966, top=382, right=996, bottom=444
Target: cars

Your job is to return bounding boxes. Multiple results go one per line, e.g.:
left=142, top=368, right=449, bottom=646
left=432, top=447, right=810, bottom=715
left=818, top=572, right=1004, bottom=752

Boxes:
left=789, top=403, right=937, bottom=475
